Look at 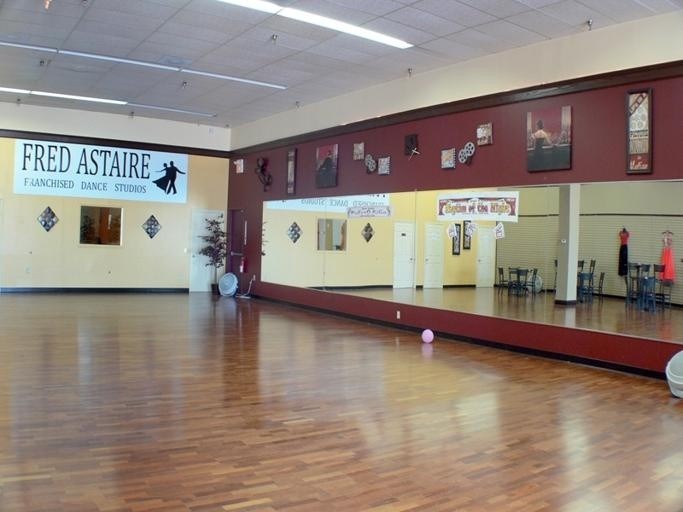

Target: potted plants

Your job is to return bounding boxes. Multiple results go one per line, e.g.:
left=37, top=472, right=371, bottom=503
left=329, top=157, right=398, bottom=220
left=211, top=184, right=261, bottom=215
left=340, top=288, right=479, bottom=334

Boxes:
left=197, top=214, right=230, bottom=295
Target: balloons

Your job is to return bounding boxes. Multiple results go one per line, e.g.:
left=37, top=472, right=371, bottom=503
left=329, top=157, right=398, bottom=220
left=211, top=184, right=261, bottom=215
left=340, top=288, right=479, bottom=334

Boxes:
left=421, top=328, right=435, bottom=344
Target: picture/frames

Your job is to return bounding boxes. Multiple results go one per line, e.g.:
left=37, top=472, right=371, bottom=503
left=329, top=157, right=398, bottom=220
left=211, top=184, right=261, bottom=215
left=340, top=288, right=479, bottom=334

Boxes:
left=286, top=147, right=297, bottom=196
left=623, top=87, right=654, bottom=174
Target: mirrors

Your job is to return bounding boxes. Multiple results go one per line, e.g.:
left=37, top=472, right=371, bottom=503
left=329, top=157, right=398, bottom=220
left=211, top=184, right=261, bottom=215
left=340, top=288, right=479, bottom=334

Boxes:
left=259, top=177, right=682, bottom=345
